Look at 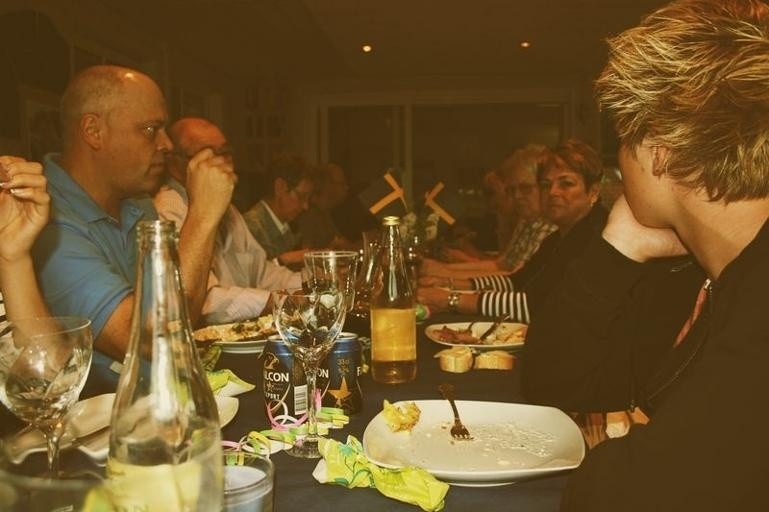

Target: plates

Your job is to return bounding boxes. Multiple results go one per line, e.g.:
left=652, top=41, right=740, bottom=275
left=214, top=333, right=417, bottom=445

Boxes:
left=426, top=321, right=529, bottom=349
left=362, top=399, right=585, bottom=488
left=185, top=320, right=265, bottom=358
left=78, top=391, right=247, bottom=461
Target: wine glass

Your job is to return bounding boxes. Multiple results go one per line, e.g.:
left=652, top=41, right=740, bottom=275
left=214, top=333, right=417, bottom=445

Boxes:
left=271, top=290, right=340, bottom=460
left=0, top=316, right=94, bottom=488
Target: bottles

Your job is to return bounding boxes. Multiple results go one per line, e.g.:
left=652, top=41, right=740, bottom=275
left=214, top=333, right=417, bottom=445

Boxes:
left=109, top=219, right=222, bottom=512
left=370, top=217, right=419, bottom=383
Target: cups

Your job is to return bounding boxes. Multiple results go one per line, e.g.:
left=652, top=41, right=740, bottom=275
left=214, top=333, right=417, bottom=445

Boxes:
left=303, top=250, right=356, bottom=315
left=352, top=231, right=423, bottom=318
left=222, top=452, right=275, bottom=512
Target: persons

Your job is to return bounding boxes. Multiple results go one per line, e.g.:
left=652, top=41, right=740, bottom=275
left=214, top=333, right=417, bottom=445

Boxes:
left=524, top=0, right=768, bottom=510
left=414, top=136, right=612, bottom=329
left=31, top=65, right=238, bottom=380
left=157, top=116, right=351, bottom=323
left=244, top=157, right=379, bottom=272
left=0, top=156, right=71, bottom=416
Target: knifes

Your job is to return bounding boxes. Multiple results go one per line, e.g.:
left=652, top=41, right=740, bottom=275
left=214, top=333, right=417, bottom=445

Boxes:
left=479, top=313, right=509, bottom=341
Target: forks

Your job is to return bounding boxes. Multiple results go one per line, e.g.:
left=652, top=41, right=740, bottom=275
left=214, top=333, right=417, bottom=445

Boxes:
left=439, top=383, right=472, bottom=442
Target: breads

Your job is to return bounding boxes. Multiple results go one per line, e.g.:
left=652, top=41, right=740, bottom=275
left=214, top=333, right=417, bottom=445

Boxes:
left=435, top=340, right=515, bottom=374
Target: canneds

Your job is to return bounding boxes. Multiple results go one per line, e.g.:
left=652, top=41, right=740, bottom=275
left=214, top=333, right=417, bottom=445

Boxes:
left=262, top=335, right=308, bottom=424
left=316, top=332, right=362, bottom=413
